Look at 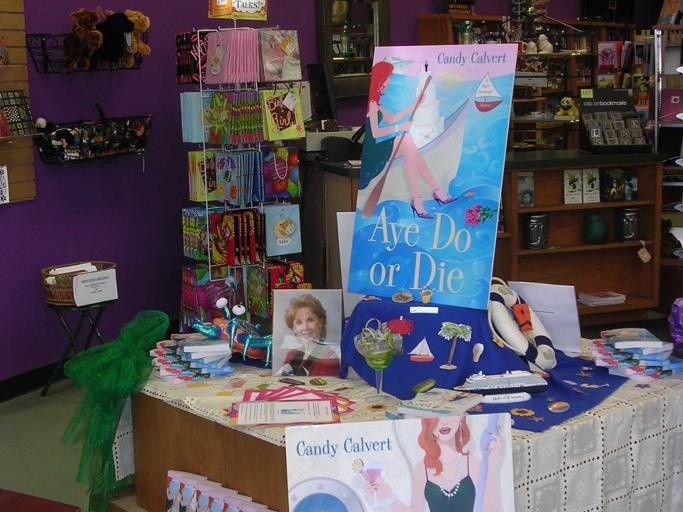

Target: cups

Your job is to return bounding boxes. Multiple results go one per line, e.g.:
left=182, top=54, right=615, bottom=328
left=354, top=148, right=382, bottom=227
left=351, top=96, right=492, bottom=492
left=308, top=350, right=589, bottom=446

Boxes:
left=511, top=41, right=528, bottom=55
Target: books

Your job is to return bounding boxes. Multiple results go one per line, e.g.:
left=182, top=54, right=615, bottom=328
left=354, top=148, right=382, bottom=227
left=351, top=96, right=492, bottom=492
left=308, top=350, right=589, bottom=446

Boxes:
left=578, top=291, right=627, bottom=307
left=587, top=328, right=683, bottom=383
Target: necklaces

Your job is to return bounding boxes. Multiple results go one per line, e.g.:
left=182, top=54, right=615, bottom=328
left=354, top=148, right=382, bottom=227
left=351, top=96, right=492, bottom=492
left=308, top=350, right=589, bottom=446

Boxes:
left=437, top=454, right=461, bottom=497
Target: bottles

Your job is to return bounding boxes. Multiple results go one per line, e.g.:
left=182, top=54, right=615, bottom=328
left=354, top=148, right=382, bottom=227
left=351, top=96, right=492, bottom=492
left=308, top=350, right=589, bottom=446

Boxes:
left=462, top=20, right=472, bottom=45
left=339, top=25, right=351, bottom=57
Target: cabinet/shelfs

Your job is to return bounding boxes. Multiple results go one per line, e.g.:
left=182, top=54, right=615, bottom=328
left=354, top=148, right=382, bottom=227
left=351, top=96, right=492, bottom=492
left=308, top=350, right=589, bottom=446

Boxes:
left=418, top=14, right=637, bottom=151
left=316, top=0, right=390, bottom=100
left=638, top=24, right=682, bottom=314
left=299, top=159, right=664, bottom=326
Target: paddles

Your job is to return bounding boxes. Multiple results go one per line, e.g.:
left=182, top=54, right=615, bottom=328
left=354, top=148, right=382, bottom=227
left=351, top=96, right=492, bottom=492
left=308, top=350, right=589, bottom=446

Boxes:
left=362, top=75, right=432, bottom=218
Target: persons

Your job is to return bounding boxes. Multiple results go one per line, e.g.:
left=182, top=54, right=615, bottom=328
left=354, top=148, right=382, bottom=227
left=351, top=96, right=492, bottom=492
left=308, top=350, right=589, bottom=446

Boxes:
left=521, top=191, right=532, bottom=203
left=610, top=178, right=632, bottom=199
left=364, top=416, right=503, bottom=511
left=357, top=62, right=457, bottom=219
left=283, top=293, right=339, bottom=376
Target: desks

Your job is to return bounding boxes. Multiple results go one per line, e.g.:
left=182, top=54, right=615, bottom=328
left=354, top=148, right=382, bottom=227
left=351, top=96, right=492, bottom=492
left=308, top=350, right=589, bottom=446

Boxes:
left=130, top=356, right=683, bottom=512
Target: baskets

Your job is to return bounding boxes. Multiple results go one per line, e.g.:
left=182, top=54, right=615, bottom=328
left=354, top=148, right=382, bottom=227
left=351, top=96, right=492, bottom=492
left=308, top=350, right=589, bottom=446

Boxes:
left=34, top=115, right=154, bottom=167
left=41, top=261, right=117, bottom=306
left=26, top=32, right=150, bottom=74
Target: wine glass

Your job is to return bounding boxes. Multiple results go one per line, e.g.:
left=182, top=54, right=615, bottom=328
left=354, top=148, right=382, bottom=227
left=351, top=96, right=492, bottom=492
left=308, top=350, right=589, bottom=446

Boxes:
left=354, top=333, right=403, bottom=404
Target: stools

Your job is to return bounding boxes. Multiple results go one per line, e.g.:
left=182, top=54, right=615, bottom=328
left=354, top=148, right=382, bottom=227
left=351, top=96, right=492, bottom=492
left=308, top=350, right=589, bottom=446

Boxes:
left=41, top=301, right=114, bottom=396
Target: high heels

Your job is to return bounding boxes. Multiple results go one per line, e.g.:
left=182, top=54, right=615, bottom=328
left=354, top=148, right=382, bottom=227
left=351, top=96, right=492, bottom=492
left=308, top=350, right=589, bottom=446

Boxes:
left=411, top=196, right=433, bottom=219
left=433, top=187, right=457, bottom=207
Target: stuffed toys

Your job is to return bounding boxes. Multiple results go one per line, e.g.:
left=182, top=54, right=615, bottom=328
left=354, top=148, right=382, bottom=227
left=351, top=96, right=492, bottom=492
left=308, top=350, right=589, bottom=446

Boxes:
left=63, top=5, right=152, bottom=70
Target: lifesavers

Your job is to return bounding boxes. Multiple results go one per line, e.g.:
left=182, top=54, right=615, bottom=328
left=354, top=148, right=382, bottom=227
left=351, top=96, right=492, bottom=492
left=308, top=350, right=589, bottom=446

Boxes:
left=489, top=282, right=558, bottom=372
